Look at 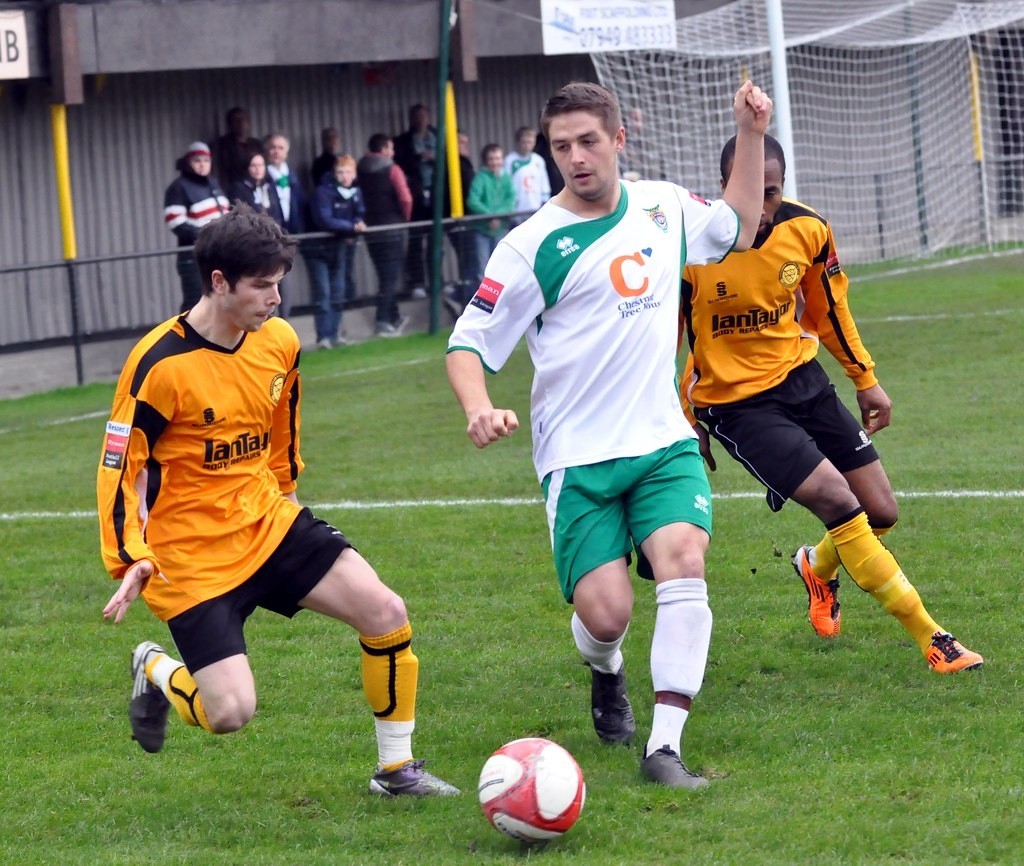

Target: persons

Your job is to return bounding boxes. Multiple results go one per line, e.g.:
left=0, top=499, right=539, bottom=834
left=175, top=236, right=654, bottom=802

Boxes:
left=260, top=135, right=302, bottom=321
left=504, top=126, right=552, bottom=230
left=216, top=104, right=260, bottom=175
left=357, top=133, right=412, bottom=338
left=311, top=131, right=345, bottom=182
left=467, top=141, right=513, bottom=289
left=443, top=129, right=475, bottom=318
left=395, top=103, right=437, bottom=299
left=445, top=75, right=774, bottom=795
left=233, top=147, right=281, bottom=244
left=165, top=139, right=230, bottom=316
left=535, top=128, right=566, bottom=197
left=96, top=197, right=467, bottom=795
left=677, top=135, right=988, bottom=675
left=299, top=151, right=366, bottom=349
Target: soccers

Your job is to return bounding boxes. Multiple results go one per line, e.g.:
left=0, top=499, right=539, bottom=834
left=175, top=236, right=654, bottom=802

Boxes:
left=478, top=737, right=587, bottom=842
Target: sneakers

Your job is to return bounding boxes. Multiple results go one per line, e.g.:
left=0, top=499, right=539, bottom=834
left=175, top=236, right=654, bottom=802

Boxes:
left=639, top=743, right=709, bottom=792
left=317, top=338, right=333, bottom=350
left=442, top=285, right=455, bottom=295
left=409, top=287, right=428, bottom=299
left=332, top=335, right=357, bottom=346
left=393, top=314, right=410, bottom=332
left=584, top=659, right=638, bottom=745
left=789, top=544, right=842, bottom=637
left=127, top=640, right=171, bottom=755
left=373, top=323, right=402, bottom=338
left=368, top=759, right=465, bottom=797
left=926, top=631, right=984, bottom=675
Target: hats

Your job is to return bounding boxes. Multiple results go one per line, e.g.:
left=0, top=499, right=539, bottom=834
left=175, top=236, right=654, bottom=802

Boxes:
left=185, top=141, right=211, bottom=157
